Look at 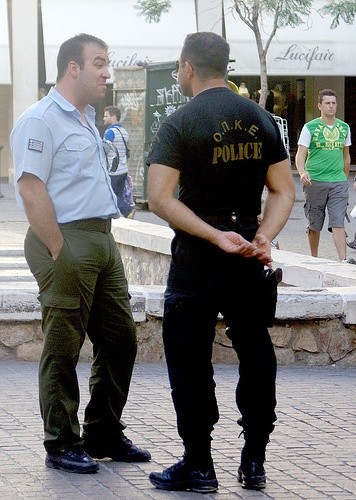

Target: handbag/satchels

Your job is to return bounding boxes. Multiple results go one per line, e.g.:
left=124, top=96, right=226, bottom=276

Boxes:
left=125, top=146, right=131, bottom=159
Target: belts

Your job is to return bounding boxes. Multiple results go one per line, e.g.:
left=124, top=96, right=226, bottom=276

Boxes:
left=73, top=216, right=113, bottom=233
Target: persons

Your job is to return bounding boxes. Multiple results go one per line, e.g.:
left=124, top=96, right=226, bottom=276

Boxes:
left=144, top=31, right=296, bottom=494
left=295, top=87, right=356, bottom=264
left=238, top=82, right=288, bottom=116
left=9, top=33, right=151, bottom=473
left=103, top=106, right=136, bottom=220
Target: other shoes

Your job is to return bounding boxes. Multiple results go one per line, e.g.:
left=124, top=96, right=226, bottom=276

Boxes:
left=340, top=258, right=356, bottom=264
left=126, top=208, right=136, bottom=219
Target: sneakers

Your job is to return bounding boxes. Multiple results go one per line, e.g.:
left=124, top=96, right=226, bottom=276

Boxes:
left=84, top=432, right=153, bottom=463
left=44, top=448, right=98, bottom=473
left=236, top=460, right=266, bottom=489
left=150, top=458, right=220, bottom=494
